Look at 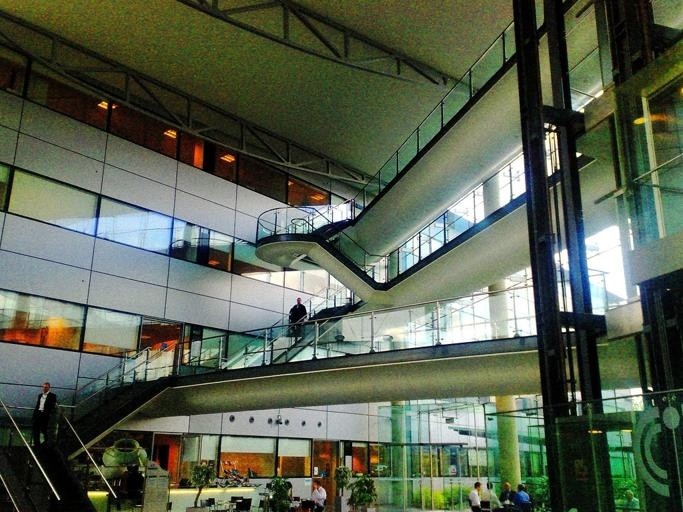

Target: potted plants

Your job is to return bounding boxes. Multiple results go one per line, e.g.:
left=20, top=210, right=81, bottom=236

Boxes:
left=333, top=465, right=380, bottom=512
left=185, top=464, right=216, bottom=512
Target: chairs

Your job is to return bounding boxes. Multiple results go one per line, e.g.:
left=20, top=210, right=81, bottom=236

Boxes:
left=199, top=492, right=327, bottom=512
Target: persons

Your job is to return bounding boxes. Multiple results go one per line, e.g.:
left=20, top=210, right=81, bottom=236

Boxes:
left=469, top=480, right=531, bottom=512
left=290, top=297, right=307, bottom=337
left=299, top=480, right=328, bottom=512
left=29, top=382, right=58, bottom=447
